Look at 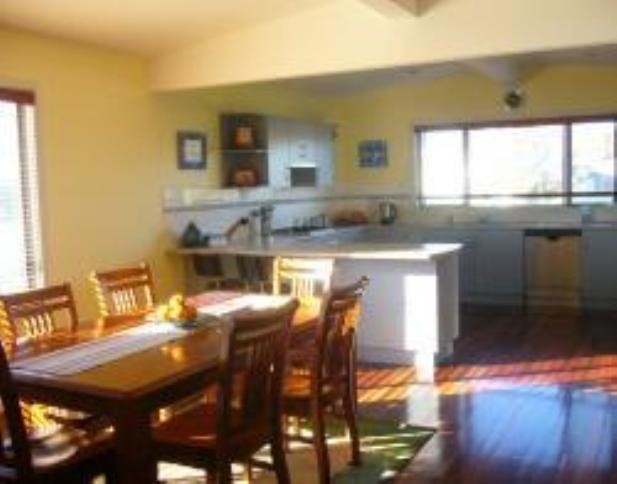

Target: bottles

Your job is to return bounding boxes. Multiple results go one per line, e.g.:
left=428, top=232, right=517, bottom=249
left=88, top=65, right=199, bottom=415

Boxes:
left=250, top=205, right=274, bottom=236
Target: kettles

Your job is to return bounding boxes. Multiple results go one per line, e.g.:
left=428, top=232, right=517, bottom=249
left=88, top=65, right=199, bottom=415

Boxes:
left=378, top=200, right=397, bottom=223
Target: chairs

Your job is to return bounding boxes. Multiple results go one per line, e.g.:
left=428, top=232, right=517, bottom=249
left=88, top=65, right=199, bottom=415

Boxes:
left=154, top=297, right=301, bottom=483
left=0, top=280, right=80, bottom=347
left=0, top=337, right=119, bottom=484
left=93, top=263, right=157, bottom=320
left=242, top=275, right=372, bottom=484
left=269, top=254, right=340, bottom=296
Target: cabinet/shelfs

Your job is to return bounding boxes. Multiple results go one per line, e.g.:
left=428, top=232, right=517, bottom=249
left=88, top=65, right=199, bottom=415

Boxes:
left=581, top=226, right=617, bottom=302
left=313, top=229, right=369, bottom=243
left=372, top=233, right=471, bottom=243
left=318, top=124, right=335, bottom=187
left=292, top=120, right=317, bottom=163
left=472, top=230, right=522, bottom=295
left=217, top=113, right=291, bottom=188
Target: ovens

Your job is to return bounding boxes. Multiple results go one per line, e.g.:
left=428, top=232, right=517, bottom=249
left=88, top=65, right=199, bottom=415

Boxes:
left=523, top=228, right=581, bottom=309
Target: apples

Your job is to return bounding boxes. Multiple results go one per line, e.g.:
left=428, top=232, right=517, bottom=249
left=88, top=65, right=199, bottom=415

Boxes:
left=154, top=292, right=195, bottom=321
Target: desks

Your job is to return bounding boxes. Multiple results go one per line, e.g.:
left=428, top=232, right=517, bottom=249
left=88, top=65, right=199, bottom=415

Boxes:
left=7, top=290, right=324, bottom=483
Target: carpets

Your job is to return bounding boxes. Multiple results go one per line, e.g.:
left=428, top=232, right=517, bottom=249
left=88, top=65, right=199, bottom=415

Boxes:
left=97, top=412, right=438, bottom=482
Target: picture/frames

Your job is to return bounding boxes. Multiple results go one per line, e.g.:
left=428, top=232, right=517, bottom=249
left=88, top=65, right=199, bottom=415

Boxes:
left=177, top=130, right=207, bottom=169
